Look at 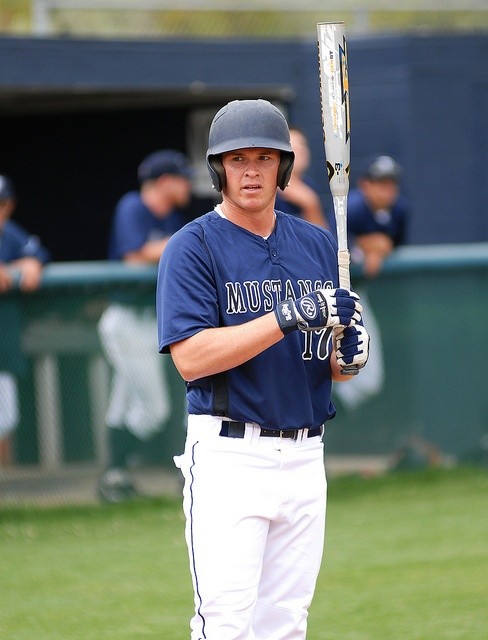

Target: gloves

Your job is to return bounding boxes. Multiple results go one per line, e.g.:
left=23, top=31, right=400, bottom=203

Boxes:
left=332, top=321, right=370, bottom=368
left=274, top=288, right=363, bottom=334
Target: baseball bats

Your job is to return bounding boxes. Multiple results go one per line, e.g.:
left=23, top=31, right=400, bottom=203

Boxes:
left=316, top=22, right=359, bottom=374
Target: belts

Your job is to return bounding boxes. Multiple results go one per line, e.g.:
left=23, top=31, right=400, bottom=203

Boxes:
left=220, top=420, right=322, bottom=438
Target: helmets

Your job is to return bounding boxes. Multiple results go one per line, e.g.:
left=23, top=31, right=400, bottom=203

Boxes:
left=205, top=98, right=295, bottom=192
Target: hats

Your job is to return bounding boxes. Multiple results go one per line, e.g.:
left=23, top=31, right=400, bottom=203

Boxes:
left=364, top=150, right=406, bottom=184
left=137, top=150, right=196, bottom=179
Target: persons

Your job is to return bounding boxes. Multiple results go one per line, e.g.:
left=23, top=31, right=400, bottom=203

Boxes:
left=0, top=175, right=52, bottom=475
left=91, top=147, right=197, bottom=505
left=270, top=127, right=328, bottom=230
left=153, top=93, right=373, bottom=640
left=328, top=154, right=413, bottom=273
